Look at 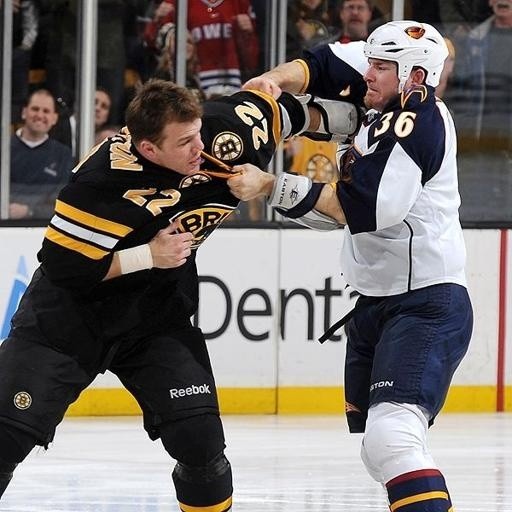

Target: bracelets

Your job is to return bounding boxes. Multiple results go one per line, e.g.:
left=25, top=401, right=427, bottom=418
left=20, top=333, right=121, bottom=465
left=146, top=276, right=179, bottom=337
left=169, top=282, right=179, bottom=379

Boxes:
left=118, top=243, right=154, bottom=275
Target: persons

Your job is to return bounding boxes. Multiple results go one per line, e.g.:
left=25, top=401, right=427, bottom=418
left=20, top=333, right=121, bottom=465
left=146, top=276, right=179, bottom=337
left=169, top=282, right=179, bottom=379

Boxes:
left=1, top=0, right=118, bottom=93
left=0, top=91, right=73, bottom=222
left=286, top=1, right=511, bottom=225
left=14, top=92, right=118, bottom=168
left=118, top=0, right=272, bottom=229
left=227, top=18, right=477, bottom=512
left=1, top=77, right=355, bottom=512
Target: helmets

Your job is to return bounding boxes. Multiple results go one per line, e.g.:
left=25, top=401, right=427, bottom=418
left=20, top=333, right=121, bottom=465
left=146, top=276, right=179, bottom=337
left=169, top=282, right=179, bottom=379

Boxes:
left=365, top=18, right=451, bottom=97
